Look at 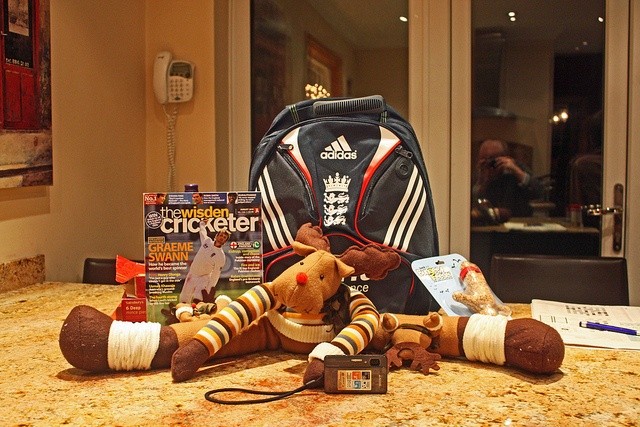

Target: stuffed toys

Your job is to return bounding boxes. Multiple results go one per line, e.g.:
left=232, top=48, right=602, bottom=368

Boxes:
left=59, top=223, right=565, bottom=383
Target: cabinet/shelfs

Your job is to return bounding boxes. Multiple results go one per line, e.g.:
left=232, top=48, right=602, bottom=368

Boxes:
left=0, top=0, right=53, bottom=190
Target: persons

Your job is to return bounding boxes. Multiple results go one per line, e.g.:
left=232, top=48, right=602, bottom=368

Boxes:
left=193, top=192, right=203, bottom=204
left=155, top=193, right=166, bottom=204
left=179, top=218, right=231, bottom=302
left=472, top=140, right=548, bottom=215
left=573, top=111, right=604, bottom=224
left=227, top=193, right=237, bottom=202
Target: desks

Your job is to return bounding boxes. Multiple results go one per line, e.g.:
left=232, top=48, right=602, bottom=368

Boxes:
left=471, top=215, right=602, bottom=284
left=0, top=254, right=640, bottom=427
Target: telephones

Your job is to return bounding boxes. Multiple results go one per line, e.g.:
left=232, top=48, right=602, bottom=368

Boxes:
left=153, top=51, right=196, bottom=191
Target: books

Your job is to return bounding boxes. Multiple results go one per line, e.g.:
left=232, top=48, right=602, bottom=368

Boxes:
left=143, top=191, right=264, bottom=327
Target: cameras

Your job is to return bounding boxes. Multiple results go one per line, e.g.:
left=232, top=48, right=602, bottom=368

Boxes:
left=323, top=354, right=388, bottom=393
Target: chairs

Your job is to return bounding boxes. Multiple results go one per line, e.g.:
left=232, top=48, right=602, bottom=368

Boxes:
left=83, top=258, right=145, bottom=285
left=489, top=253, right=630, bottom=304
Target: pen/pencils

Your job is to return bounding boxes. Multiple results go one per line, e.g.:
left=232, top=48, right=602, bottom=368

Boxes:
left=525, top=222, right=542, bottom=226
left=579, top=320, right=640, bottom=335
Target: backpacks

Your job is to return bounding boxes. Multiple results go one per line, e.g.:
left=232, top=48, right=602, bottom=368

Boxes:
left=248, top=95, right=441, bottom=316
